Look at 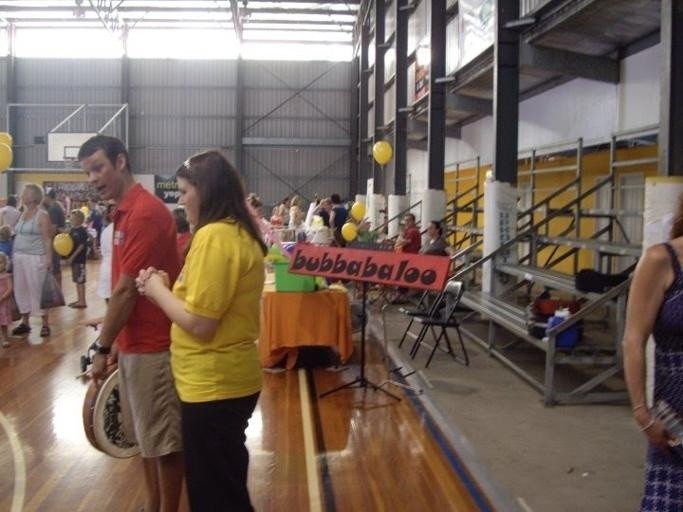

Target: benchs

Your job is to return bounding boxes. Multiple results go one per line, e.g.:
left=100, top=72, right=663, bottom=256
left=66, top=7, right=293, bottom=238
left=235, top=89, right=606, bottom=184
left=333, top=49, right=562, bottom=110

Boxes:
left=371, top=186, right=643, bottom=409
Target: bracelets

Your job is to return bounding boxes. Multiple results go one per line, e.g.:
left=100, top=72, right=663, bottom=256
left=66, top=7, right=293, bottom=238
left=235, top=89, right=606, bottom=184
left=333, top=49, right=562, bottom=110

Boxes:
left=89, top=340, right=112, bottom=355
left=633, top=401, right=644, bottom=410
left=637, top=418, right=658, bottom=430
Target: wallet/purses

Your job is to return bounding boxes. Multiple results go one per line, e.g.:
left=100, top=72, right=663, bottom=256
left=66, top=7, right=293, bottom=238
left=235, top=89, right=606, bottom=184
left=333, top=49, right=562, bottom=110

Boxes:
left=647, top=400, right=682, bottom=456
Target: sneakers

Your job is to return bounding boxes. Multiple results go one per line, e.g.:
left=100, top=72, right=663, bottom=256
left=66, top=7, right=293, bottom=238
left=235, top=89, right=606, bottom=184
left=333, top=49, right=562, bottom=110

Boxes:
left=40, top=326, right=49, bottom=337
left=12, top=324, right=31, bottom=335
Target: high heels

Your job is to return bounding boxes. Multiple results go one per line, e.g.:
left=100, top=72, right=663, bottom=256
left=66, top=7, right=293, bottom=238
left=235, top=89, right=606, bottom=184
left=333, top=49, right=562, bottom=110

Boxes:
left=2, top=337, right=10, bottom=348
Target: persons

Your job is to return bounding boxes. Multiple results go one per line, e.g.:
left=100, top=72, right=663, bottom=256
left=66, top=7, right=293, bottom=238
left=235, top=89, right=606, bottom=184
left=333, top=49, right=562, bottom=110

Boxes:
left=131, top=150, right=268, bottom=511
left=171, top=184, right=456, bottom=306
left=78, top=133, right=186, bottom=510
left=620, top=189, right=682, bottom=511
left=0, top=184, right=119, bottom=348
